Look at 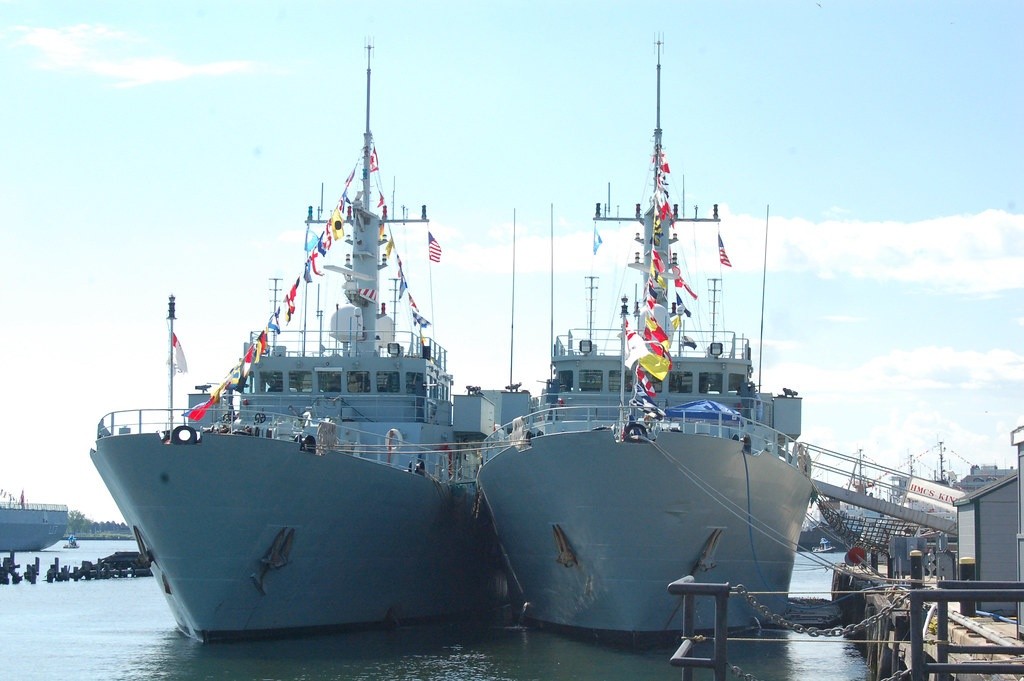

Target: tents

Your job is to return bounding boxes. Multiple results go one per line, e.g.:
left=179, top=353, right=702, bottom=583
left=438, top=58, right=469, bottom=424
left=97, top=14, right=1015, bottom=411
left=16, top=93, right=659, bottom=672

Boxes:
left=664, top=399, right=744, bottom=427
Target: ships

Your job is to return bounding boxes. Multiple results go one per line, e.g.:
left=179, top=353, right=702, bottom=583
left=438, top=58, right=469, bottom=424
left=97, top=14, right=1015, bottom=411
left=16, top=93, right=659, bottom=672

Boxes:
left=475, top=28, right=816, bottom=654
left=88, top=33, right=497, bottom=647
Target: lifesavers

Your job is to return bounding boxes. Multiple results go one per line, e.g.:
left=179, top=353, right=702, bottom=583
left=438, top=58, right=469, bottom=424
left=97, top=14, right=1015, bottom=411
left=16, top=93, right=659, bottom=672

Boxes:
left=621, top=422, right=648, bottom=444
left=384, top=428, right=403, bottom=453
left=173, top=424, right=197, bottom=444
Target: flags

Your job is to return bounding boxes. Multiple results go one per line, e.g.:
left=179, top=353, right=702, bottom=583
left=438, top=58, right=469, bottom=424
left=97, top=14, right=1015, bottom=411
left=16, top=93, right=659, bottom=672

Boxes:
left=171, top=331, right=188, bottom=374
left=429, top=230, right=442, bottom=262
left=624, top=144, right=700, bottom=418
left=181, top=141, right=431, bottom=420
left=594, top=228, right=602, bottom=255
left=718, top=232, right=732, bottom=267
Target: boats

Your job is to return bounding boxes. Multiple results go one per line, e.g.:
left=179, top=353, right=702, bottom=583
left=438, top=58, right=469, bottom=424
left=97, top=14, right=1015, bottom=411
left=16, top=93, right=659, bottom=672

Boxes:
left=812, top=537, right=836, bottom=553
left=62, top=535, right=80, bottom=548
left=0, top=490, right=70, bottom=552
